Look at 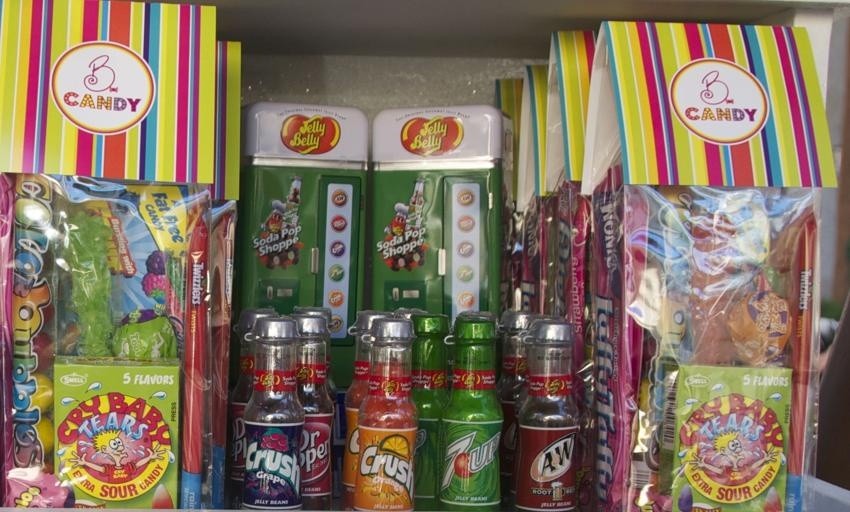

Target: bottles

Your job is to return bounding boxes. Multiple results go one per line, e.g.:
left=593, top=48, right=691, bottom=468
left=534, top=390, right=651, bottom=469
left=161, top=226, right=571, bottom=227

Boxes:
left=343, top=312, right=387, bottom=512
left=514, top=322, right=577, bottom=512
left=243, top=316, right=303, bottom=509
left=408, top=313, right=451, bottom=512
left=496, top=313, right=542, bottom=512
left=229, top=308, right=278, bottom=484
left=290, top=308, right=335, bottom=466
left=437, top=312, right=501, bottom=511
left=355, top=320, right=418, bottom=511
left=287, top=316, right=331, bottom=510
left=396, top=308, right=426, bottom=324
